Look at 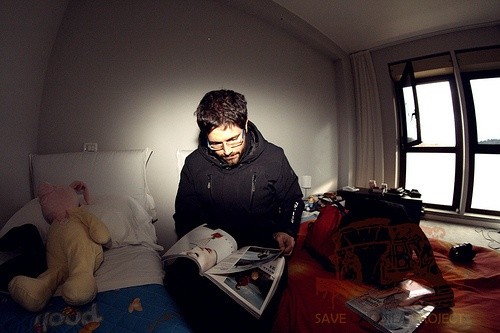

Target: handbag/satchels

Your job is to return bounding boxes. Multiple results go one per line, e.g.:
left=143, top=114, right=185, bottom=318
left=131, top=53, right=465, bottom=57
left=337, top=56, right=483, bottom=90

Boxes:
left=301, top=189, right=440, bottom=289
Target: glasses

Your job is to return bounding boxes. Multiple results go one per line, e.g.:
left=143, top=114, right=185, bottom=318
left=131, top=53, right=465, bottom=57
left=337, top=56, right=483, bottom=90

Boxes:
left=205, top=139, right=246, bottom=151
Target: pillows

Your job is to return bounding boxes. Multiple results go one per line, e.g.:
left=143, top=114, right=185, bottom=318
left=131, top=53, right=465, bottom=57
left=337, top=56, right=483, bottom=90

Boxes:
left=28, top=147, right=154, bottom=198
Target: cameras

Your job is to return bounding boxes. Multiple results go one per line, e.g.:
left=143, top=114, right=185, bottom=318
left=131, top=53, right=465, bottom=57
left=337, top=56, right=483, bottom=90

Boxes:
left=82, top=143, right=97, bottom=152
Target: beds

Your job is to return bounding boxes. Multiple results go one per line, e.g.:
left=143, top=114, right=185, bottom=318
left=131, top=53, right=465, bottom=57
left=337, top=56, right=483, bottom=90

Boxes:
left=272, top=212, right=500, bottom=333
left=0, top=197, right=190, bottom=333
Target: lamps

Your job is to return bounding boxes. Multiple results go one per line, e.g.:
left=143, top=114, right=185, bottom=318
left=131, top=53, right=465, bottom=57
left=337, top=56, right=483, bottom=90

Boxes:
left=301, top=176, right=311, bottom=198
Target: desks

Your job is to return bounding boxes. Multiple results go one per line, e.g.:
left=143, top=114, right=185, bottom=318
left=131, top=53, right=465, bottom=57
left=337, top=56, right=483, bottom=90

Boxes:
left=336, top=187, right=422, bottom=225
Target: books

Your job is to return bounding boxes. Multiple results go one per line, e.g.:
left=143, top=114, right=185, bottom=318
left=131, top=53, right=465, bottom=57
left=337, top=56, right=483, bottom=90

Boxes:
left=345, top=279, right=434, bottom=333
left=163, top=223, right=285, bottom=320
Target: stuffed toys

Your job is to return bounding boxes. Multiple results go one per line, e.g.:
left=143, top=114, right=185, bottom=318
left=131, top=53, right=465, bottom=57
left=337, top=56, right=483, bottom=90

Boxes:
left=8, top=181, right=111, bottom=312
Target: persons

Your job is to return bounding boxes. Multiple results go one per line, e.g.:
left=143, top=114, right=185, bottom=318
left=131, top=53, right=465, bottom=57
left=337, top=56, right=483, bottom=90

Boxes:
left=236, top=276, right=252, bottom=290
left=251, top=271, right=258, bottom=280
left=163, top=89, right=305, bottom=333
